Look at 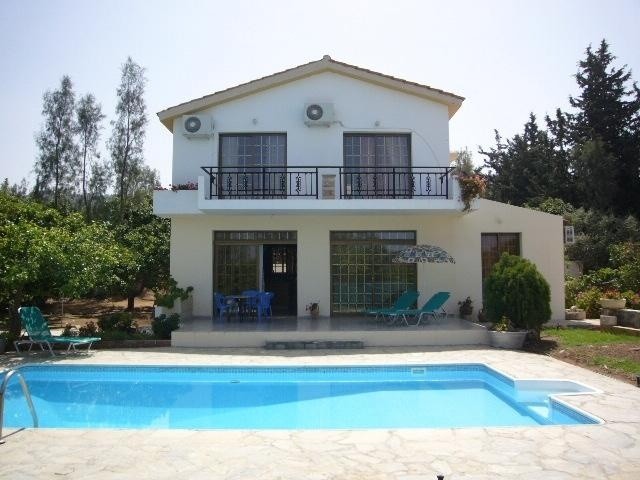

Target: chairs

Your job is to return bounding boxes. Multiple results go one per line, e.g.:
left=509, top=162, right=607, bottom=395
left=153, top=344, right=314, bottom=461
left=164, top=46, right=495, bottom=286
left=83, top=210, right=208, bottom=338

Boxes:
left=12, top=304, right=102, bottom=355
left=363, top=288, right=453, bottom=327
left=209, top=286, right=276, bottom=327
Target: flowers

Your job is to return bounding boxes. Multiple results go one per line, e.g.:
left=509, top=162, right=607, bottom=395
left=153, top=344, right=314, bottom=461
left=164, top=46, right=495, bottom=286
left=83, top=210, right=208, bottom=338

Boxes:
left=458, top=170, right=489, bottom=216
left=153, top=180, right=199, bottom=193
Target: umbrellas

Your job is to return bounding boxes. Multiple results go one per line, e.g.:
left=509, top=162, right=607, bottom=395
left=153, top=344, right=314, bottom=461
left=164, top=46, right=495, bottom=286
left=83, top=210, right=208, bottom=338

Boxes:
left=391, top=242, right=457, bottom=305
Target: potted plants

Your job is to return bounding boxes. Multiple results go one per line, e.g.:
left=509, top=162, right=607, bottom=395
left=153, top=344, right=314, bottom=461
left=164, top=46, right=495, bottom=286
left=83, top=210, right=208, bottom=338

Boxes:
left=303, top=294, right=322, bottom=321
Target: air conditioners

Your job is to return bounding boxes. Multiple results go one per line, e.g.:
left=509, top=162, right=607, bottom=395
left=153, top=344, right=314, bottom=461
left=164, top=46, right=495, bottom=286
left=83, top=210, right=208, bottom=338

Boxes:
left=178, top=112, right=217, bottom=142
left=303, top=100, right=338, bottom=128
left=561, top=224, right=577, bottom=246
